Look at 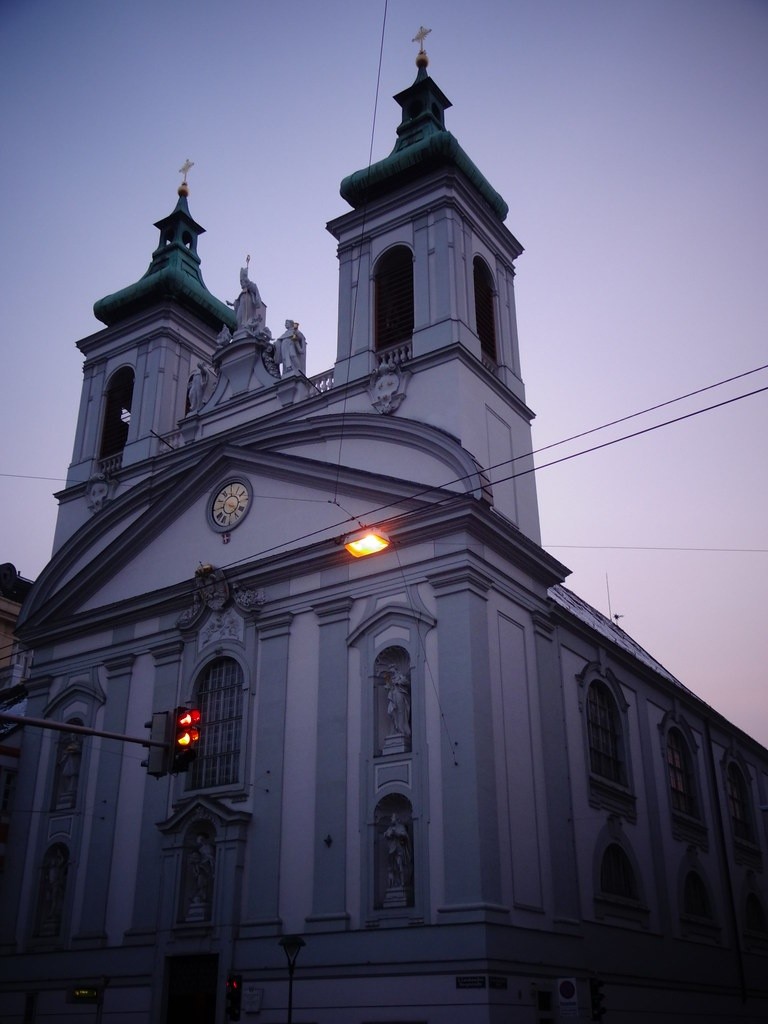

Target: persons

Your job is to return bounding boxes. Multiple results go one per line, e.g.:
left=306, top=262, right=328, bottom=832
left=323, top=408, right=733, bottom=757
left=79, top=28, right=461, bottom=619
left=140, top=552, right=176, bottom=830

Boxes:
left=188, top=835, right=214, bottom=903
left=226, top=267, right=266, bottom=330
left=44, top=850, right=64, bottom=918
left=383, top=812, right=412, bottom=887
left=57, top=719, right=83, bottom=792
left=386, top=664, right=411, bottom=736
left=186, top=361, right=209, bottom=412
left=272, top=319, right=307, bottom=375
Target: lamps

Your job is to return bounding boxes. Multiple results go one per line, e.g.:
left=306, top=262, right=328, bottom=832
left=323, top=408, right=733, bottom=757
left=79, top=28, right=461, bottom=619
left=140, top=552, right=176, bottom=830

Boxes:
left=343, top=528, right=391, bottom=558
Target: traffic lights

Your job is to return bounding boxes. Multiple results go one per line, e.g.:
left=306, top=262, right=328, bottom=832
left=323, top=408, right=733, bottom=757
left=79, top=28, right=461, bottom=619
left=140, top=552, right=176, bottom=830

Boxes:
left=140, top=711, right=168, bottom=776
left=225, top=974, right=241, bottom=1020
left=170, top=704, right=203, bottom=770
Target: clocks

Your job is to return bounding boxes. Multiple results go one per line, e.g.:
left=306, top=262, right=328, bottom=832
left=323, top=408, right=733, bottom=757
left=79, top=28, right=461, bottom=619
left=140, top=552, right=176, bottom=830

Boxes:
left=205, top=474, right=253, bottom=534
